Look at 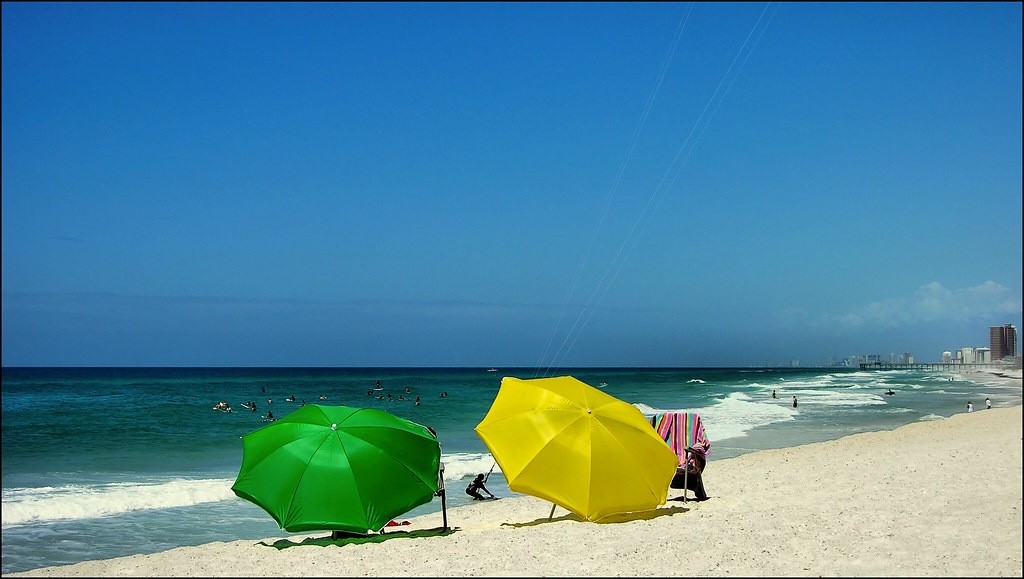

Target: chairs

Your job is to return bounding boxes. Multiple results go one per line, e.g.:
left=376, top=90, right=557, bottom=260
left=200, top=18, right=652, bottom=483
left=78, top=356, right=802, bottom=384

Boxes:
left=649, top=412, right=710, bottom=504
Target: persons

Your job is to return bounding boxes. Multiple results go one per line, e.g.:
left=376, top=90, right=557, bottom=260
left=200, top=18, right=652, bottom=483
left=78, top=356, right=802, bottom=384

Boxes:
left=379, top=393, right=406, bottom=401
left=268, top=398, right=273, bottom=403
left=405, top=385, right=411, bottom=393
left=967, top=401, right=973, bottom=412
left=320, top=395, right=325, bottom=399
left=244, top=401, right=256, bottom=411
left=465, top=474, right=494, bottom=500
left=793, top=396, right=798, bottom=407
left=440, top=391, right=448, bottom=397
left=375, top=380, right=382, bottom=389
left=368, top=388, right=373, bottom=395
left=290, top=395, right=295, bottom=401
left=215, top=401, right=231, bottom=413
left=415, top=395, right=420, bottom=405
left=772, top=390, right=776, bottom=398
left=267, top=411, right=273, bottom=418
left=985, top=398, right=991, bottom=409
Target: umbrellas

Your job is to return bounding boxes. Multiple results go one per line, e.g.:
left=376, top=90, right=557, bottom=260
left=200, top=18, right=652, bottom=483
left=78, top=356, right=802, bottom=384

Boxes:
left=473, top=375, right=678, bottom=523
left=233, top=404, right=440, bottom=536
